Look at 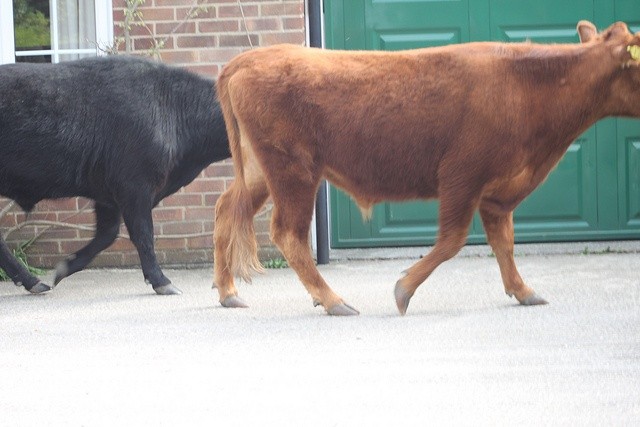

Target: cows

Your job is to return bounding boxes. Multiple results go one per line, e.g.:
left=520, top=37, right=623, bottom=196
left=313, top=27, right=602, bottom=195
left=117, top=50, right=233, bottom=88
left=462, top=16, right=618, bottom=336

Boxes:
left=212, top=20, right=640, bottom=319
left=0, top=51, right=222, bottom=296
